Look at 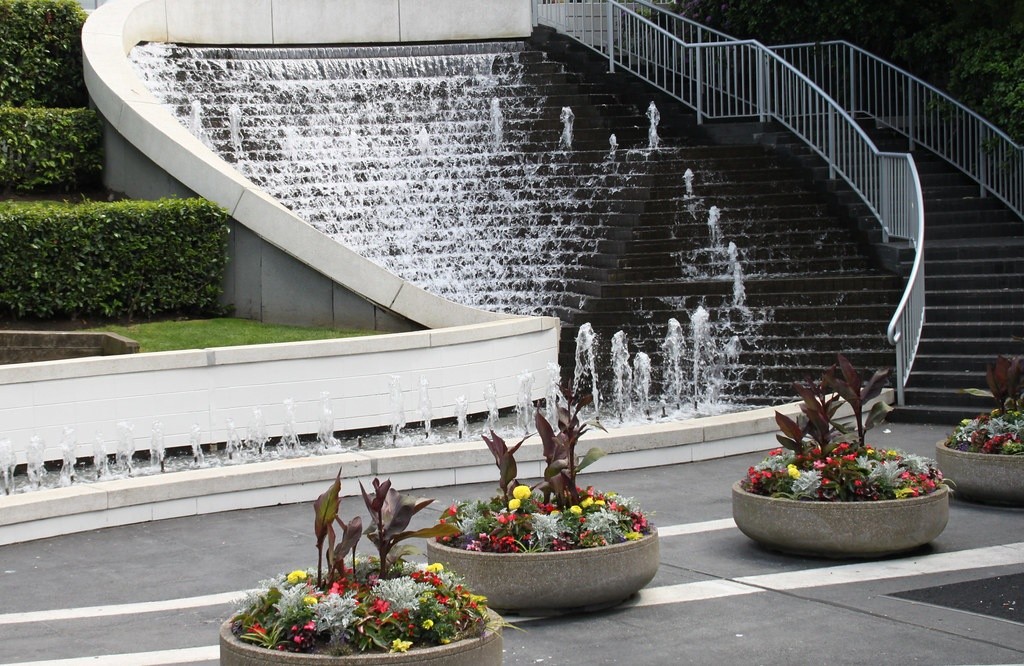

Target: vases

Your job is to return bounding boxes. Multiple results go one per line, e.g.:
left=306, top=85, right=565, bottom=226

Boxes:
left=935, top=438, right=1024, bottom=510
left=732, top=480, right=949, bottom=560
left=219, top=607, right=503, bottom=666
left=425, top=528, right=660, bottom=616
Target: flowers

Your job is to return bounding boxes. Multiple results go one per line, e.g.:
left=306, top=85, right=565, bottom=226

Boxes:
left=944, top=352, right=1024, bottom=455
left=740, top=351, right=954, bottom=502
left=434, top=377, right=656, bottom=554
left=228, top=467, right=526, bottom=655
